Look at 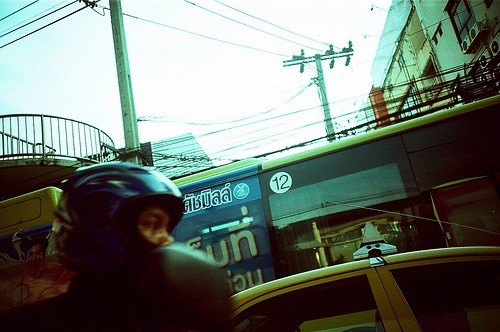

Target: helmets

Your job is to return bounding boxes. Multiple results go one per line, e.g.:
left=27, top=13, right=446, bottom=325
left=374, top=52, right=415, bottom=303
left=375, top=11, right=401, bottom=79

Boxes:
left=52, top=161, right=185, bottom=280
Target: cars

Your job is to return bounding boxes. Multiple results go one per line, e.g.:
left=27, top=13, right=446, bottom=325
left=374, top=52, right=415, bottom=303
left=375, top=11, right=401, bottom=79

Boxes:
left=230, top=221, right=500, bottom=332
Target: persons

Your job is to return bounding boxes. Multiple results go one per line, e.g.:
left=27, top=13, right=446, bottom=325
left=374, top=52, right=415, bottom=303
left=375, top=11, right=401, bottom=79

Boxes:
left=0, top=161, right=233, bottom=331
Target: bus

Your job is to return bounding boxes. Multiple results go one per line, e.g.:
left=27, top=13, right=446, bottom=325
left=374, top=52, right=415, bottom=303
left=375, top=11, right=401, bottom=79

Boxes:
left=1, top=94, right=500, bottom=310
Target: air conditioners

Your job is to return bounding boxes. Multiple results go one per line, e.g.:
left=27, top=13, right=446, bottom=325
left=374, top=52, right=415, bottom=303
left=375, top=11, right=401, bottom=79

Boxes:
left=489, top=32, right=500, bottom=56
left=460, top=36, right=477, bottom=54
left=478, top=48, right=491, bottom=69
left=469, top=22, right=489, bottom=43
